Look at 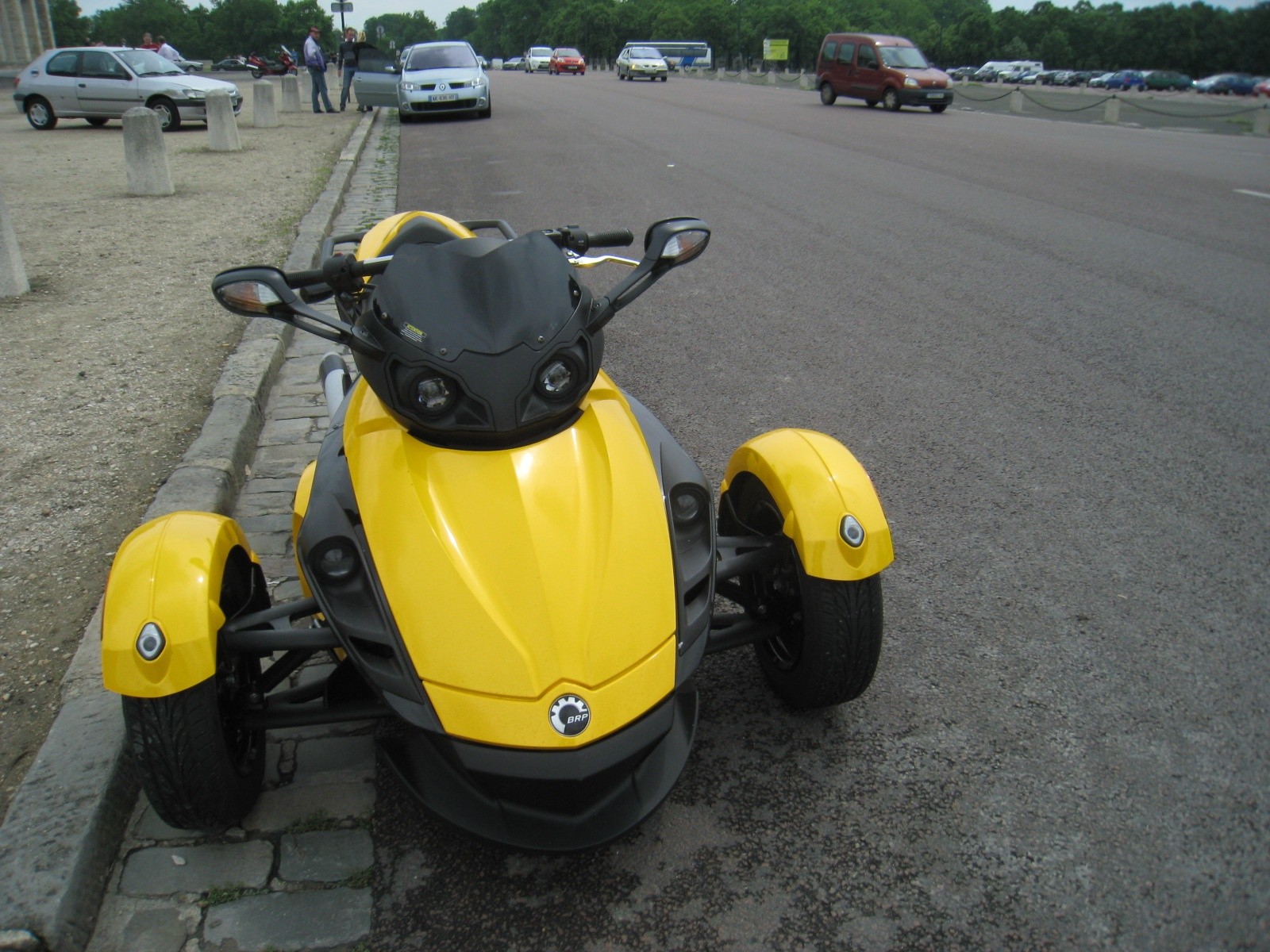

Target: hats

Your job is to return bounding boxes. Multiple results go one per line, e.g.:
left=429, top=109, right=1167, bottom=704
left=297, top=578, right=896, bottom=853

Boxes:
left=310, top=26, right=322, bottom=32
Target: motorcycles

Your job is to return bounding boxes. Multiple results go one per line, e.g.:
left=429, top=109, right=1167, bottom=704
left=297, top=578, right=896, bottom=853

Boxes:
left=101, top=212, right=897, bottom=855
left=246, top=45, right=298, bottom=79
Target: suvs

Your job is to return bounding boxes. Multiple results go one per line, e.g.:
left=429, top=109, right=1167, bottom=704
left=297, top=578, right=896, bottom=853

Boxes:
left=524, top=47, right=554, bottom=73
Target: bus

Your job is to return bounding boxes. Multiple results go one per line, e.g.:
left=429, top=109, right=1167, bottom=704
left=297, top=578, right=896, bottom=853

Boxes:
left=622, top=42, right=712, bottom=72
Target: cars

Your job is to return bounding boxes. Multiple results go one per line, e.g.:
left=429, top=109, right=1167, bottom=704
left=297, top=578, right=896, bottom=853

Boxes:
left=171, top=56, right=203, bottom=73
left=477, top=56, right=503, bottom=70
left=503, top=57, right=526, bottom=70
left=946, top=67, right=979, bottom=82
left=1193, top=74, right=1270, bottom=99
left=616, top=47, right=668, bottom=82
left=12, top=47, right=243, bottom=130
left=211, top=59, right=248, bottom=72
left=548, top=48, right=585, bottom=76
left=1004, top=70, right=1107, bottom=87
left=1088, top=69, right=1193, bottom=92
left=353, top=42, right=491, bottom=123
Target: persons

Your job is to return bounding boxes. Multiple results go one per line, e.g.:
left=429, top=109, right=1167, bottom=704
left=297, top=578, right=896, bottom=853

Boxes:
left=85, top=38, right=96, bottom=47
left=303, top=26, right=341, bottom=113
left=157, top=35, right=186, bottom=64
left=96, top=40, right=106, bottom=47
left=337, top=27, right=366, bottom=113
left=352, top=42, right=378, bottom=111
left=121, top=39, right=126, bottom=47
left=290, top=48, right=298, bottom=67
left=140, top=33, right=160, bottom=53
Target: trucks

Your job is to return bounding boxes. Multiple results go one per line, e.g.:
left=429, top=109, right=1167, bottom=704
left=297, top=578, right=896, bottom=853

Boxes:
left=974, top=61, right=1012, bottom=80
left=999, top=60, right=1044, bottom=79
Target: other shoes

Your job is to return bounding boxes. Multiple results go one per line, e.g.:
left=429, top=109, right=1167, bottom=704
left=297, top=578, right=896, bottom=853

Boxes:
left=366, top=108, right=373, bottom=111
left=341, top=107, right=345, bottom=111
left=357, top=107, right=364, bottom=111
left=327, top=108, right=339, bottom=113
left=315, top=109, right=324, bottom=113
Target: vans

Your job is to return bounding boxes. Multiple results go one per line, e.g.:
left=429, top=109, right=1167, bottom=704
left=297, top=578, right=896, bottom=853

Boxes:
left=815, top=33, right=954, bottom=114
